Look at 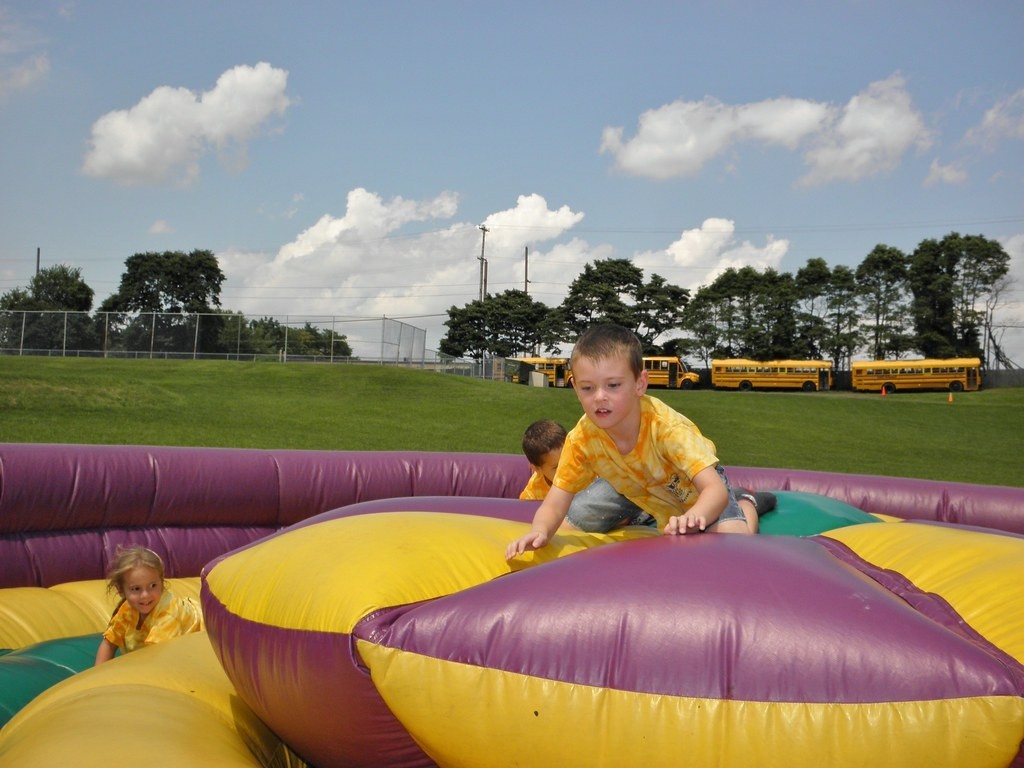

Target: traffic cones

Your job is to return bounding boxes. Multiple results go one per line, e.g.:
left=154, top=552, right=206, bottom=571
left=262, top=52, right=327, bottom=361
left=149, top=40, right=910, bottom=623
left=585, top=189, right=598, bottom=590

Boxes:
left=948, top=393, right=954, bottom=402
left=882, top=387, right=886, bottom=396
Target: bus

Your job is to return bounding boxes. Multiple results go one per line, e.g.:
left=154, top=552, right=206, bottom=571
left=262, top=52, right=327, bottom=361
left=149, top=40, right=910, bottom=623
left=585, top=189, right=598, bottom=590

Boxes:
left=851, top=357, right=983, bottom=394
left=711, top=359, right=833, bottom=393
left=642, top=356, right=700, bottom=390
left=492, top=358, right=574, bottom=388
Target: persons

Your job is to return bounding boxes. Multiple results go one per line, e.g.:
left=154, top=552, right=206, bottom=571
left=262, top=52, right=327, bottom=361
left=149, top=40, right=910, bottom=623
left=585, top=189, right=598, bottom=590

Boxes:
left=504, top=322, right=778, bottom=563
left=95, top=543, right=206, bottom=666
left=520, top=418, right=569, bottom=501
